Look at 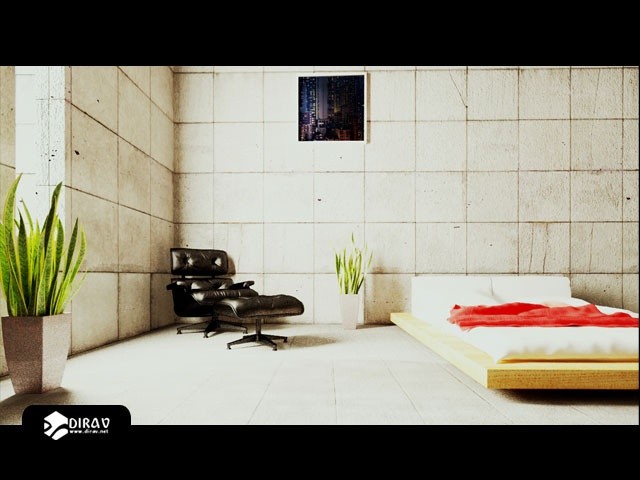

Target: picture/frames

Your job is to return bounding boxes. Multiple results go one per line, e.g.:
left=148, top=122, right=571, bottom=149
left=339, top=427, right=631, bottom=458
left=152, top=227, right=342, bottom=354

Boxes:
left=292, top=70, right=370, bottom=146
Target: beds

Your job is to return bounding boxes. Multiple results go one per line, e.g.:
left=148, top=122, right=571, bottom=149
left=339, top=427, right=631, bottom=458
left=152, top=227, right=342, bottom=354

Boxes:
left=389, top=271, right=640, bottom=393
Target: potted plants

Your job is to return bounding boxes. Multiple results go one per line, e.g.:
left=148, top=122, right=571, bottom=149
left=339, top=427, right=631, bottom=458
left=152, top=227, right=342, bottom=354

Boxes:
left=1, top=171, right=89, bottom=396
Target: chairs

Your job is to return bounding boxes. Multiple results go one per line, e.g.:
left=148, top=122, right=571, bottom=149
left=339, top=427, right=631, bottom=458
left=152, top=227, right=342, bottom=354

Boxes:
left=165, top=247, right=305, bottom=352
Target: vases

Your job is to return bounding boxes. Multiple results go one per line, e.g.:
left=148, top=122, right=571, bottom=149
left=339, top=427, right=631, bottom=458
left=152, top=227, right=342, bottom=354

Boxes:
left=334, top=230, right=376, bottom=331
